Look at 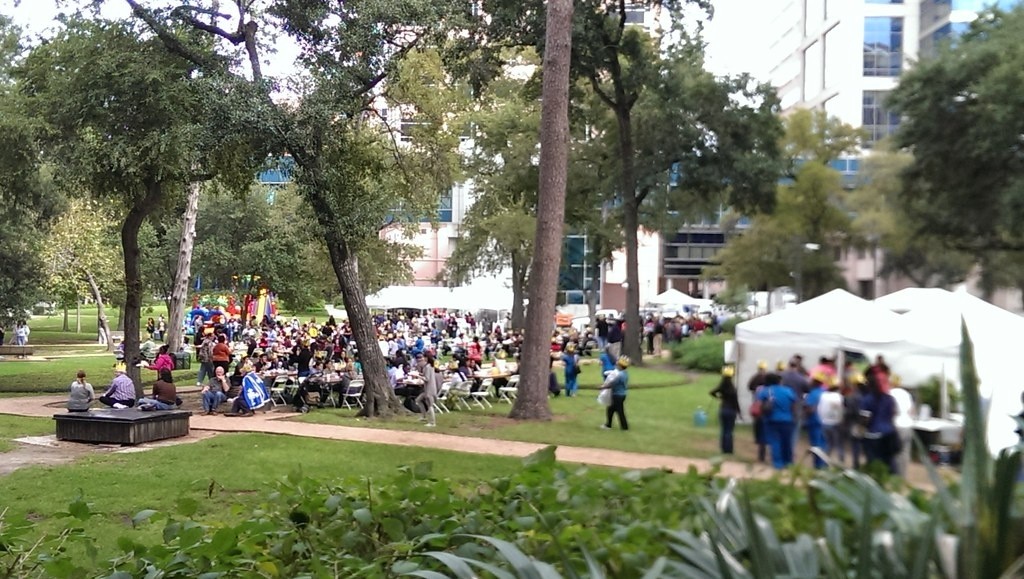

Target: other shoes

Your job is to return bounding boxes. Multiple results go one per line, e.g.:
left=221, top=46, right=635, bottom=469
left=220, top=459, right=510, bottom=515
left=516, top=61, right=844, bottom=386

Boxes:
left=600, top=424, right=611, bottom=429
left=225, top=413, right=236, bottom=416
left=210, top=411, right=217, bottom=415
left=242, top=412, right=254, bottom=417
left=201, top=410, right=210, bottom=416
left=424, top=422, right=436, bottom=426
left=177, top=401, right=182, bottom=406
left=420, top=417, right=428, bottom=420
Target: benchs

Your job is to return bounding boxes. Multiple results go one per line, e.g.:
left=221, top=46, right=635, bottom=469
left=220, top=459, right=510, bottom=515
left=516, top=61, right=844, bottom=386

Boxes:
left=112, top=330, right=142, bottom=343
left=52, top=407, right=193, bottom=445
left=0, top=344, right=34, bottom=359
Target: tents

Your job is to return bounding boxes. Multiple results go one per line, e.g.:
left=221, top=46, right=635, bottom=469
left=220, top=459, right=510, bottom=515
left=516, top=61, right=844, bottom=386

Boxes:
left=360, top=267, right=530, bottom=337
left=644, top=287, right=1024, bottom=462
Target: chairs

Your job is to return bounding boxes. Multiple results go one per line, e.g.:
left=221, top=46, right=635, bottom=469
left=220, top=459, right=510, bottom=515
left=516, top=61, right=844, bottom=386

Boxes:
left=341, top=380, right=365, bottom=410
left=267, top=374, right=287, bottom=407
left=470, top=377, right=493, bottom=409
left=583, top=341, right=596, bottom=357
left=447, top=381, right=472, bottom=410
left=497, top=374, right=519, bottom=405
left=427, top=381, right=451, bottom=414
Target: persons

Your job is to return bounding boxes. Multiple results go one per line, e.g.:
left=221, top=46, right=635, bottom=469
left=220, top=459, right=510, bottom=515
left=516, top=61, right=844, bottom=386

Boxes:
left=65, top=314, right=183, bottom=412
left=0, top=318, right=31, bottom=359
left=710, top=353, right=916, bottom=478
left=187, top=309, right=718, bottom=431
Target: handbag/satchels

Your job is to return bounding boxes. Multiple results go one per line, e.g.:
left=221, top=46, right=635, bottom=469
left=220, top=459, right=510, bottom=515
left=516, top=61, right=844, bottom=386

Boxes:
left=573, top=365, right=581, bottom=373
left=764, top=388, right=775, bottom=413
left=750, top=387, right=762, bottom=418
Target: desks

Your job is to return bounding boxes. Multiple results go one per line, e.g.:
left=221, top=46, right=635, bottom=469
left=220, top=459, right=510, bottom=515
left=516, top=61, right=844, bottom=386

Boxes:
left=266, top=371, right=297, bottom=377
left=467, top=369, right=511, bottom=378
left=323, top=377, right=341, bottom=407
left=398, top=379, right=425, bottom=408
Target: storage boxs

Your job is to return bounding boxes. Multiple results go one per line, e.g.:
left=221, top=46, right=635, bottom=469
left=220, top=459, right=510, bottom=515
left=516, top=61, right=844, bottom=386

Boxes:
left=590, top=349, right=600, bottom=357
